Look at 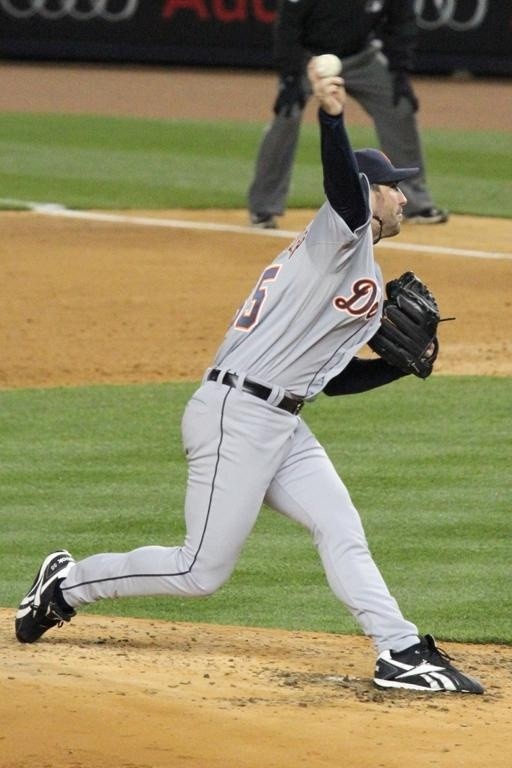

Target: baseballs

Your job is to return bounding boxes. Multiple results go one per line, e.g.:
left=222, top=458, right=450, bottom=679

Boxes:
left=316, top=54, right=341, bottom=78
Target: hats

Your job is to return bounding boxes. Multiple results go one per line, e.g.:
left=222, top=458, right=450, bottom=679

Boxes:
left=347, top=148, right=421, bottom=182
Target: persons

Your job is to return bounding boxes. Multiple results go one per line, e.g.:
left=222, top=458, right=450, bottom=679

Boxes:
left=246, top=1, right=448, bottom=229
left=15, top=53, right=488, bottom=695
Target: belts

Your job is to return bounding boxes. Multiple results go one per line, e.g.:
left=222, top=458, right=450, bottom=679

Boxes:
left=202, top=365, right=305, bottom=415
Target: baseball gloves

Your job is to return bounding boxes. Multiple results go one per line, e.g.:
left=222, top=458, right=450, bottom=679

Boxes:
left=366, top=270, right=455, bottom=380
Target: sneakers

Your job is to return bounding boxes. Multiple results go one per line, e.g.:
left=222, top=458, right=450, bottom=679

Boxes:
left=245, top=207, right=275, bottom=229
left=14, top=547, right=77, bottom=643
left=404, top=206, right=449, bottom=228
left=373, top=635, right=485, bottom=695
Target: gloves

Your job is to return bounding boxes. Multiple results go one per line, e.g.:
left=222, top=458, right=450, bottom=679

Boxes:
left=390, top=71, right=420, bottom=114
left=272, top=73, right=307, bottom=117
